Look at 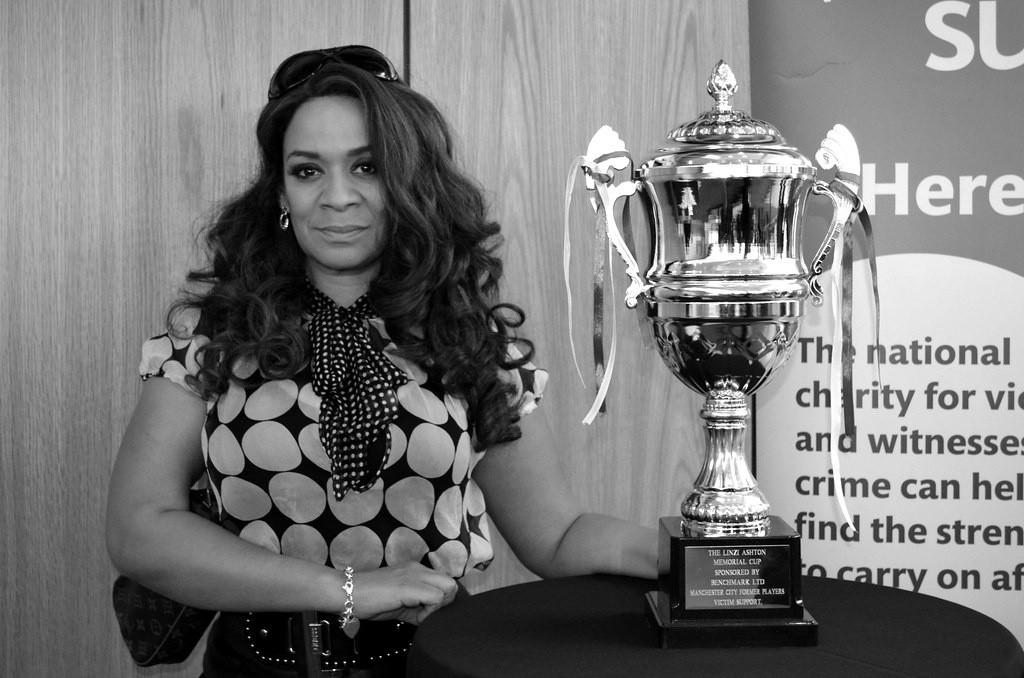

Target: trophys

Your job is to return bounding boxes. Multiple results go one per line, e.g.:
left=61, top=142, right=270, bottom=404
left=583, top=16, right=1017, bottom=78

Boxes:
left=562, top=60, right=881, bottom=644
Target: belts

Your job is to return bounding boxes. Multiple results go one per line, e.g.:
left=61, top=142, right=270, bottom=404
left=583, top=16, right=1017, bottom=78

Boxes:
left=231, top=607, right=422, bottom=678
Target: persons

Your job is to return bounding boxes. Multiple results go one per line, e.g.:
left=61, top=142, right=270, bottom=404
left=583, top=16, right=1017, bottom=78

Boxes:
left=679, top=325, right=765, bottom=390
left=106, top=45, right=658, bottom=678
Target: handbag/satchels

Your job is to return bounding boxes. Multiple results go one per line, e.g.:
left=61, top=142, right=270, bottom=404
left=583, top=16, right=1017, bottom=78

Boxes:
left=111, top=487, right=223, bottom=668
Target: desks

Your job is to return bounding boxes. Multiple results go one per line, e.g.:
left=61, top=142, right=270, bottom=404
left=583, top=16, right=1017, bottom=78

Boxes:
left=402, top=572, right=1024, bottom=678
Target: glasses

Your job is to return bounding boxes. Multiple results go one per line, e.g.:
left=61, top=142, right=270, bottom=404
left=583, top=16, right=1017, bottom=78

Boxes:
left=268, top=44, right=404, bottom=107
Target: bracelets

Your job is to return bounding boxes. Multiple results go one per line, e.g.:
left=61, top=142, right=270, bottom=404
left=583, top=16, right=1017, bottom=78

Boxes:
left=339, top=566, right=357, bottom=628
